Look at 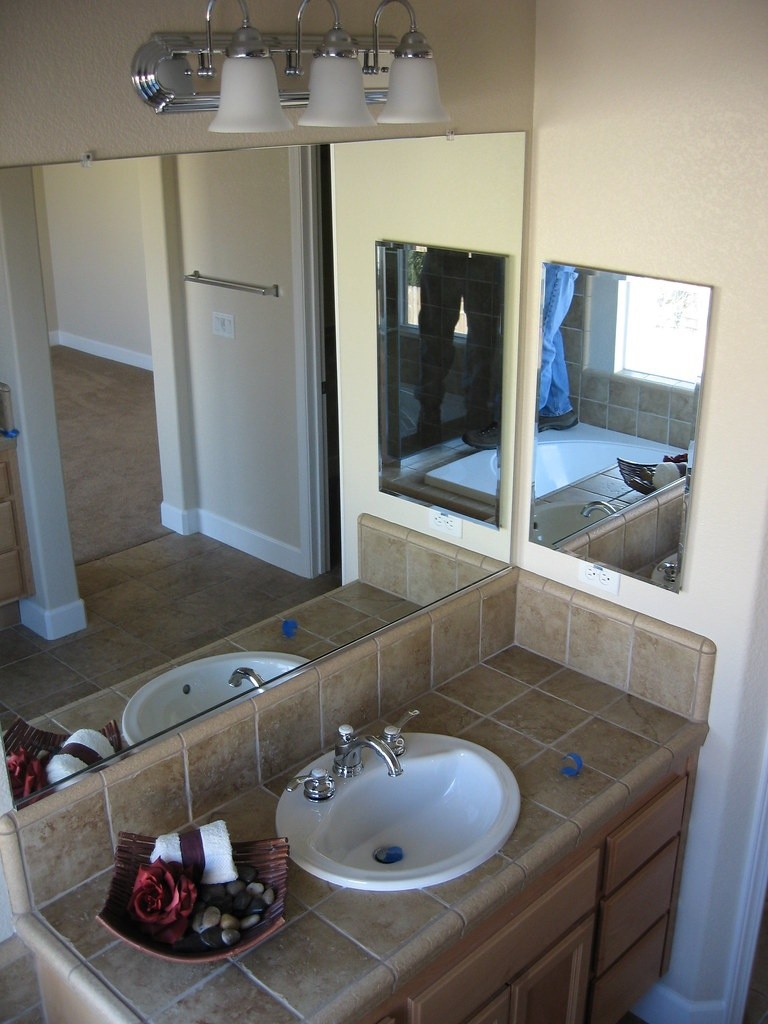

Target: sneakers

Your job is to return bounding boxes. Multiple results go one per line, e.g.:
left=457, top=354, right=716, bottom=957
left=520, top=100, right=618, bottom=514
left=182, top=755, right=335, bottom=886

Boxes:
left=463, top=423, right=502, bottom=450
left=537, top=410, right=577, bottom=432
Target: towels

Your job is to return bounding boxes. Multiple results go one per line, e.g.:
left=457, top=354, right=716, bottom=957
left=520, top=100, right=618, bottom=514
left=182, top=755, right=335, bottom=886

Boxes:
left=150, top=820, right=238, bottom=885
left=47, top=728, right=116, bottom=791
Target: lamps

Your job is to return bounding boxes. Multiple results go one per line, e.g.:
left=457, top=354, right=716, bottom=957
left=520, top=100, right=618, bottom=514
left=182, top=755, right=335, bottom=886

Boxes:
left=129, top=1, right=450, bottom=135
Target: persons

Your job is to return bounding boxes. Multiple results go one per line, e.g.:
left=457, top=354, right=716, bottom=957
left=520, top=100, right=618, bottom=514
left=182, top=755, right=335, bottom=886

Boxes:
left=407, top=246, right=580, bottom=450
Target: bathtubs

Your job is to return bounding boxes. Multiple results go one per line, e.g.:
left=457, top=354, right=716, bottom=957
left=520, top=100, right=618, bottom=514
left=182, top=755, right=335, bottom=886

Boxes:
left=425, top=420, right=689, bottom=506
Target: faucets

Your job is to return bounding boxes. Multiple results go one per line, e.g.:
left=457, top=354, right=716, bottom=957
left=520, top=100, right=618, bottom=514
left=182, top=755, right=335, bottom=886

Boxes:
left=228, top=667, right=267, bottom=694
left=580, top=500, right=616, bottom=518
left=332, top=724, right=403, bottom=778
left=657, top=562, right=678, bottom=582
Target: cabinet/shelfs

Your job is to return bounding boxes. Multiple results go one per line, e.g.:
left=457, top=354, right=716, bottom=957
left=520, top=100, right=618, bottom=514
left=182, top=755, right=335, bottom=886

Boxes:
left=403, top=772, right=691, bottom=1023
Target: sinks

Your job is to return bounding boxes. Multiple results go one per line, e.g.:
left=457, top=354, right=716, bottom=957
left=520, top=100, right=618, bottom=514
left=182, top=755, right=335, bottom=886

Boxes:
left=274, top=732, right=522, bottom=893
left=531, top=502, right=608, bottom=544
left=121, top=651, right=312, bottom=747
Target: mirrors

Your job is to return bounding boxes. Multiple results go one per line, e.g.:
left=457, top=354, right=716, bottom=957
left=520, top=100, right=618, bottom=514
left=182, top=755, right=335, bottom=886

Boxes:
left=526, top=261, right=713, bottom=594
left=373, top=239, right=511, bottom=531
left=0, top=124, right=526, bottom=809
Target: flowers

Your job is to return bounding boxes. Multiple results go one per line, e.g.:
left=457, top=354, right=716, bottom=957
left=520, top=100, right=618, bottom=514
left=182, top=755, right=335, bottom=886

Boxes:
left=126, top=855, right=199, bottom=951
left=5, top=746, right=49, bottom=805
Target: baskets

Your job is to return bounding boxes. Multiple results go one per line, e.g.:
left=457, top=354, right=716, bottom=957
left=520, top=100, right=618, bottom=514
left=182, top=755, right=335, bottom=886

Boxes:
left=4, top=716, right=120, bottom=765
left=96, top=830, right=289, bottom=963
left=616, top=457, right=663, bottom=495
